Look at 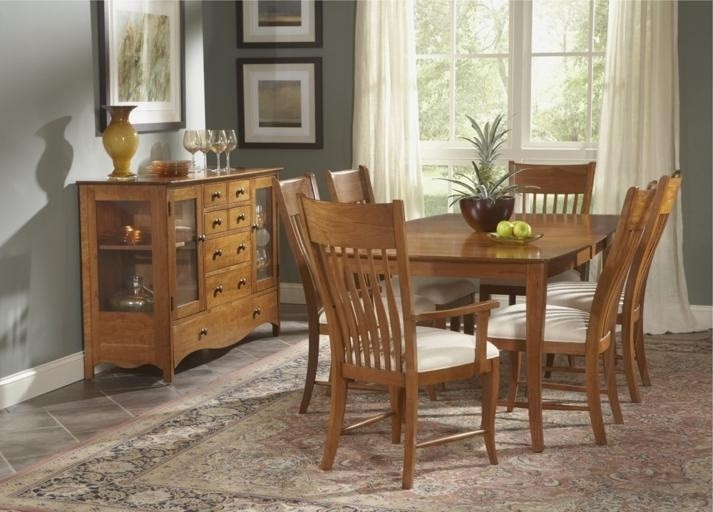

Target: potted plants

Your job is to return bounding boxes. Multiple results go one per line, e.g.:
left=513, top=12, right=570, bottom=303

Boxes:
left=433, top=113, right=540, bottom=232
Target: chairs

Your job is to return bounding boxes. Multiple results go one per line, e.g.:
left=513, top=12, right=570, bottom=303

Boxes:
left=487, top=180, right=661, bottom=443
left=545, top=170, right=683, bottom=402
left=272, top=172, right=445, bottom=415
left=296, top=193, right=499, bottom=488
left=479, top=161, right=597, bottom=306
left=324, top=166, right=477, bottom=336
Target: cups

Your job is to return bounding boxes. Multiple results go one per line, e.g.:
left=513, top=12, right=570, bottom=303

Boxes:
left=207, top=129, right=227, bottom=174
left=182, top=129, right=201, bottom=174
left=218, top=129, right=237, bottom=172
left=197, top=130, right=212, bottom=172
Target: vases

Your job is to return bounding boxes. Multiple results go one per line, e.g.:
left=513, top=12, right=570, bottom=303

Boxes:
left=102, top=105, right=138, bottom=176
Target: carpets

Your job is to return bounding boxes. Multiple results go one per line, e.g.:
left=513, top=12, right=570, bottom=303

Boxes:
left=0, top=330, right=712, bottom=511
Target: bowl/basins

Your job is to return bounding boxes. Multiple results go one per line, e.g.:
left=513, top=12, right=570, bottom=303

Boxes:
left=150, top=160, right=192, bottom=179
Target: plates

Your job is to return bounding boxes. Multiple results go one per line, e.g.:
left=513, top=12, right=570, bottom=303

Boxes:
left=485, top=231, right=545, bottom=247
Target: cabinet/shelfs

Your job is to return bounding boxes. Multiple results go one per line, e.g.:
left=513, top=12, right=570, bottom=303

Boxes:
left=77, top=166, right=283, bottom=384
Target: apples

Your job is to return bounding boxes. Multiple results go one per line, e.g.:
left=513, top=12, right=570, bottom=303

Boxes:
left=513, top=222, right=531, bottom=240
left=497, top=220, right=514, bottom=237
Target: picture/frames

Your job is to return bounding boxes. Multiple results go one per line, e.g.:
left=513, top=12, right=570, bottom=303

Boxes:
left=98, top=1, right=186, bottom=132
left=237, top=58, right=322, bottom=149
left=236, top=0, right=323, bottom=48
